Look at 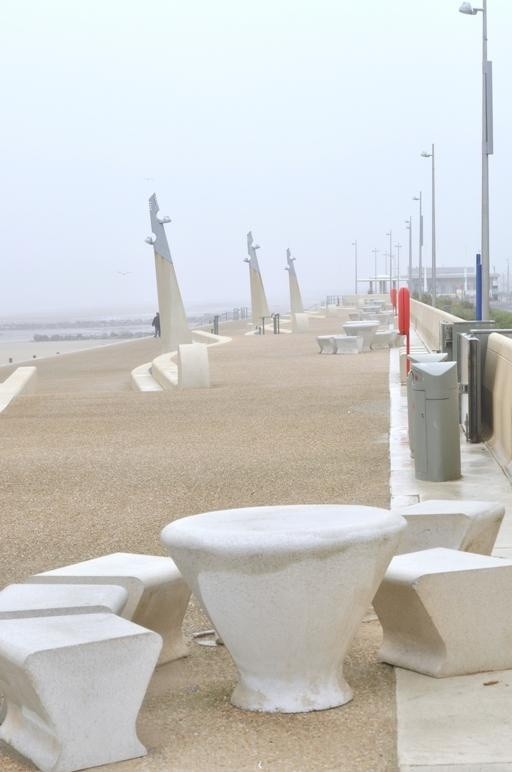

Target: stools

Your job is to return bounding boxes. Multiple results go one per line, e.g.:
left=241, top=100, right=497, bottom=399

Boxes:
left=317, top=334, right=362, bottom=355
left=370, top=328, right=405, bottom=350
left=373, top=496, right=511, bottom=679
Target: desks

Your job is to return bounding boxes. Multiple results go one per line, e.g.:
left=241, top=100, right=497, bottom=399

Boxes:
left=164, top=504, right=408, bottom=718
left=343, top=323, right=380, bottom=352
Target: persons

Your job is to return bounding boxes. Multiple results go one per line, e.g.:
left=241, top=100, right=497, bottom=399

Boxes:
left=152, top=312, right=160, bottom=336
